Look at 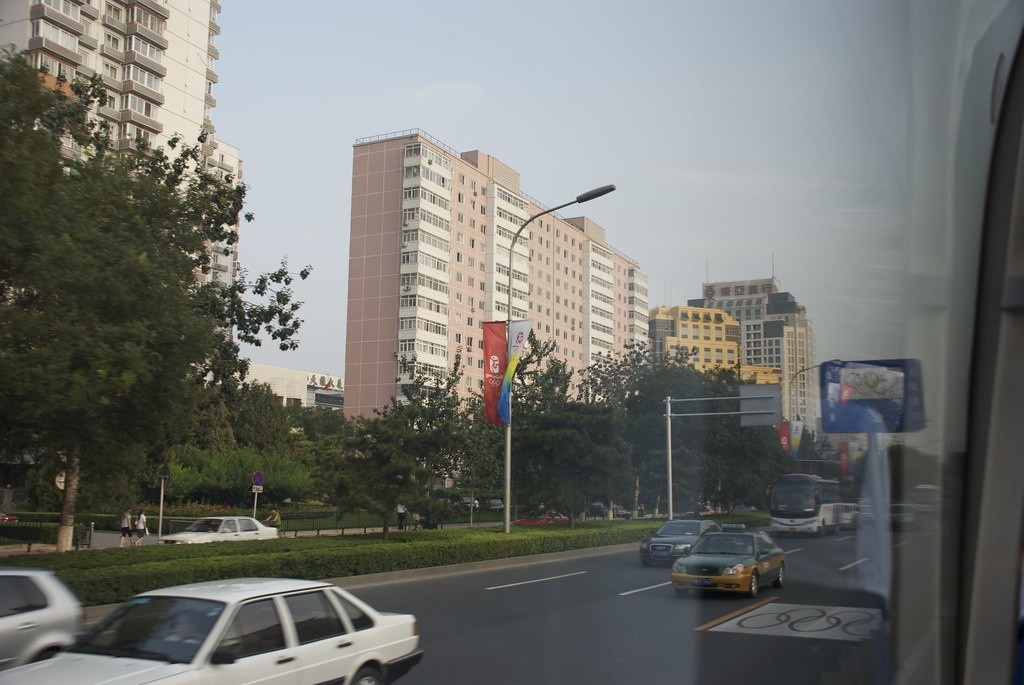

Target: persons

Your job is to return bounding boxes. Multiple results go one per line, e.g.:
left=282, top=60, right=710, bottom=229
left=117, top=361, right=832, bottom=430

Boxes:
left=395, top=504, right=408, bottom=530
left=264, top=508, right=281, bottom=528
left=120, top=507, right=133, bottom=547
left=134, top=509, right=146, bottom=546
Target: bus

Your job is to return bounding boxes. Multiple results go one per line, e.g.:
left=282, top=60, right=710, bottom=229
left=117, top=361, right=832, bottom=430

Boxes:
left=766, top=474, right=844, bottom=539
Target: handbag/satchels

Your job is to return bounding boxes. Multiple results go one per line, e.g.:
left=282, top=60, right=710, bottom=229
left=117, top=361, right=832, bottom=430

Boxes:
left=144, top=527, right=149, bottom=536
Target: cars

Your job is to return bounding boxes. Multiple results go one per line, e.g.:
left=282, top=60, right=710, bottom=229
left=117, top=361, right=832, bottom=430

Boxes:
left=158, top=516, right=280, bottom=544
left=0, top=577, right=425, bottom=685
left=511, top=503, right=569, bottom=526
left=839, top=503, right=923, bottom=531
left=640, top=518, right=718, bottom=567
left=671, top=524, right=788, bottom=599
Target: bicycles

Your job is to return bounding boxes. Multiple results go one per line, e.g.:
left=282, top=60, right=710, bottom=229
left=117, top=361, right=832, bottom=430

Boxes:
left=263, top=520, right=286, bottom=538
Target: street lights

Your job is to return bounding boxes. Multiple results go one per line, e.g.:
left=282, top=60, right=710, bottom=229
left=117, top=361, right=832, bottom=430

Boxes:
left=503, top=186, right=615, bottom=533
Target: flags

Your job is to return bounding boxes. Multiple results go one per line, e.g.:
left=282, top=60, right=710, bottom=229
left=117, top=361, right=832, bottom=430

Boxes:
left=482, top=321, right=508, bottom=426
left=780, top=421, right=804, bottom=462
left=840, top=442, right=858, bottom=477
left=498, top=320, right=533, bottom=424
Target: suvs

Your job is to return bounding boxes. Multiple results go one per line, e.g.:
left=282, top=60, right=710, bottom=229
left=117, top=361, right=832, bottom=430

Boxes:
left=0, top=567, right=88, bottom=671
